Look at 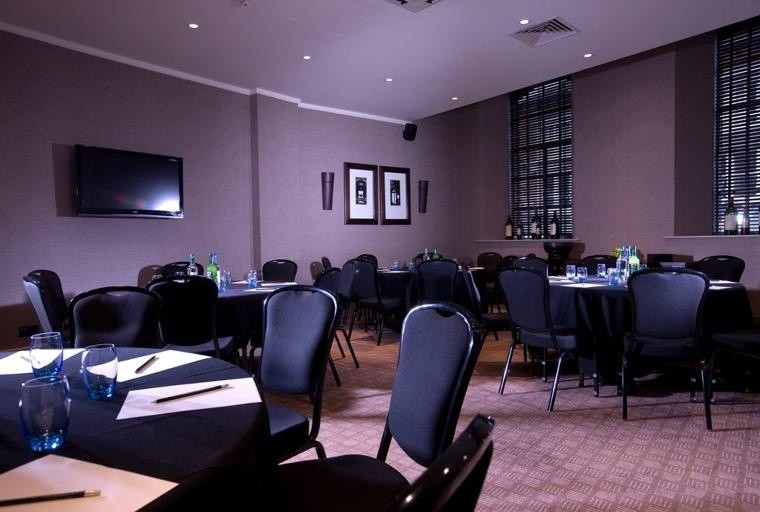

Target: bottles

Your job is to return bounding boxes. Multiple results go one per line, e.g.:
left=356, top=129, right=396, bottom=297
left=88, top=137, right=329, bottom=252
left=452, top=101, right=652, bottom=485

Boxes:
left=615, top=242, right=640, bottom=283
left=423, top=248, right=440, bottom=261
left=724, top=197, right=738, bottom=235
left=504, top=209, right=559, bottom=240
left=187, top=249, right=220, bottom=292
left=741, top=208, right=750, bottom=235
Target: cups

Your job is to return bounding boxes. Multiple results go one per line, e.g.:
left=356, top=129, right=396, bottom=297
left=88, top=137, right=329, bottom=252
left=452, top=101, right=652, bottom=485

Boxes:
left=220, top=270, right=257, bottom=293
left=176, top=272, right=185, bottom=275
left=82, top=344, right=118, bottom=400
left=29, top=332, right=63, bottom=381
left=18, top=376, right=71, bottom=452
left=391, top=256, right=422, bottom=272
left=566, top=264, right=619, bottom=288
left=152, top=275, right=162, bottom=281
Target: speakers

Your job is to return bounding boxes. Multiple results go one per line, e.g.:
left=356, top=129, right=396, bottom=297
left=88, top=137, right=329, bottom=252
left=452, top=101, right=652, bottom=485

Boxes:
left=405, top=123, right=417, bottom=141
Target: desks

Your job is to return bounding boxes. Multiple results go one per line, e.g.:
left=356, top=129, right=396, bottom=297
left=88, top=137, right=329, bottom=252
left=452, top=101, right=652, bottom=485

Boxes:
left=1, top=347, right=270, bottom=511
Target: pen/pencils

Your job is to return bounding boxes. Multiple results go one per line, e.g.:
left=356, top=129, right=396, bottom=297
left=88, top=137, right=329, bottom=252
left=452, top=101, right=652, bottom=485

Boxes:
left=150, top=383, right=230, bottom=405
left=136, top=354, right=158, bottom=374
left=0, top=489, right=101, bottom=507
left=21, top=355, right=33, bottom=364
left=256, top=288, right=276, bottom=290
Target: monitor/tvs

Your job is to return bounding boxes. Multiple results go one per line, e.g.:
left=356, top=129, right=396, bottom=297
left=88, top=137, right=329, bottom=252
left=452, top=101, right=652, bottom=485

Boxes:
left=73, top=144, right=185, bottom=220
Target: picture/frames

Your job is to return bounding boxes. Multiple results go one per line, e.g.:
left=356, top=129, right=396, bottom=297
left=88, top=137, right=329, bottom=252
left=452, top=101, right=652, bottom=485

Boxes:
left=343, top=162, right=378, bottom=225
left=379, top=166, right=411, bottom=225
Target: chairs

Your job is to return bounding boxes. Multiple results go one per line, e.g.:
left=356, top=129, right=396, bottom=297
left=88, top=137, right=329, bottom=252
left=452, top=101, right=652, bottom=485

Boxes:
left=261, top=302, right=495, bottom=511
left=257, top=286, right=340, bottom=464
